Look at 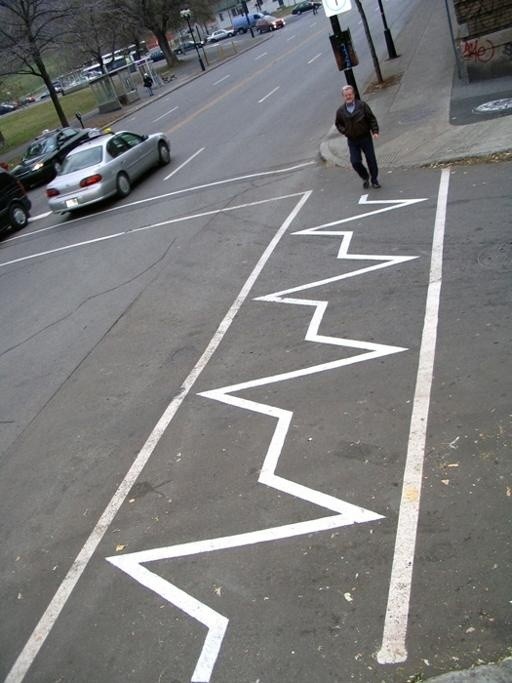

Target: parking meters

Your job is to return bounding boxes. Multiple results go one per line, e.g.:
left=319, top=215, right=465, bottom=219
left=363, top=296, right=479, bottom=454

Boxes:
left=75, top=111, right=85, bottom=129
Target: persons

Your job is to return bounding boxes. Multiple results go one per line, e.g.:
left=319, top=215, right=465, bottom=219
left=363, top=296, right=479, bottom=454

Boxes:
left=335, top=85, right=383, bottom=189
left=143, top=73, right=154, bottom=96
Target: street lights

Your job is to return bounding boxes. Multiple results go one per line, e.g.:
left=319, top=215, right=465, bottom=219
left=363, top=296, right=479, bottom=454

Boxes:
left=179, top=8, right=206, bottom=71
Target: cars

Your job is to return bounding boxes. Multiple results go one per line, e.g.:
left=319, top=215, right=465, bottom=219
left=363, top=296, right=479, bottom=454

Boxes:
left=0, top=71, right=102, bottom=114
left=9, top=124, right=92, bottom=191
left=149, top=27, right=238, bottom=62
left=46, top=127, right=171, bottom=215
left=291, top=0, right=322, bottom=15
left=255, top=15, right=286, bottom=34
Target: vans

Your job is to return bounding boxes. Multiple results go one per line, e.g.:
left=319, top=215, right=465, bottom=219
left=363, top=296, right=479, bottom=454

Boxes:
left=231, top=11, right=272, bottom=35
left=0, top=161, right=31, bottom=239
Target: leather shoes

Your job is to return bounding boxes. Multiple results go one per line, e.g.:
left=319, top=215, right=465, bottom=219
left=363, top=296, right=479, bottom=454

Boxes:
left=364, top=182, right=369, bottom=189
left=372, top=184, right=381, bottom=188
